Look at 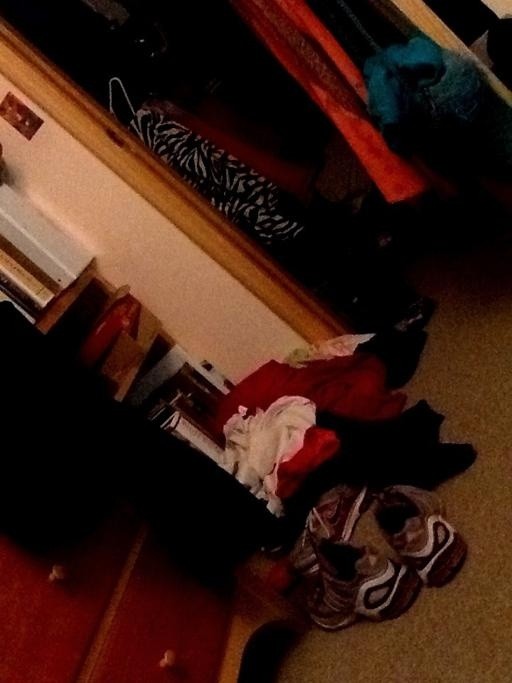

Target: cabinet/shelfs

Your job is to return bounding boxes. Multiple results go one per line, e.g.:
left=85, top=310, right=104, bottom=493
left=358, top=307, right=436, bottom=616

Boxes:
left=0, top=256, right=292, bottom=682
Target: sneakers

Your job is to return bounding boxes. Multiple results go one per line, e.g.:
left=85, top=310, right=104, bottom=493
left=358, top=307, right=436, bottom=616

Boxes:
left=372, top=483, right=467, bottom=587
left=308, top=535, right=420, bottom=631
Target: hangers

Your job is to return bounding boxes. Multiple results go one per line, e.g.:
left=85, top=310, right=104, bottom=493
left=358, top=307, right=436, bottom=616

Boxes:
left=108, top=77, right=134, bottom=118
